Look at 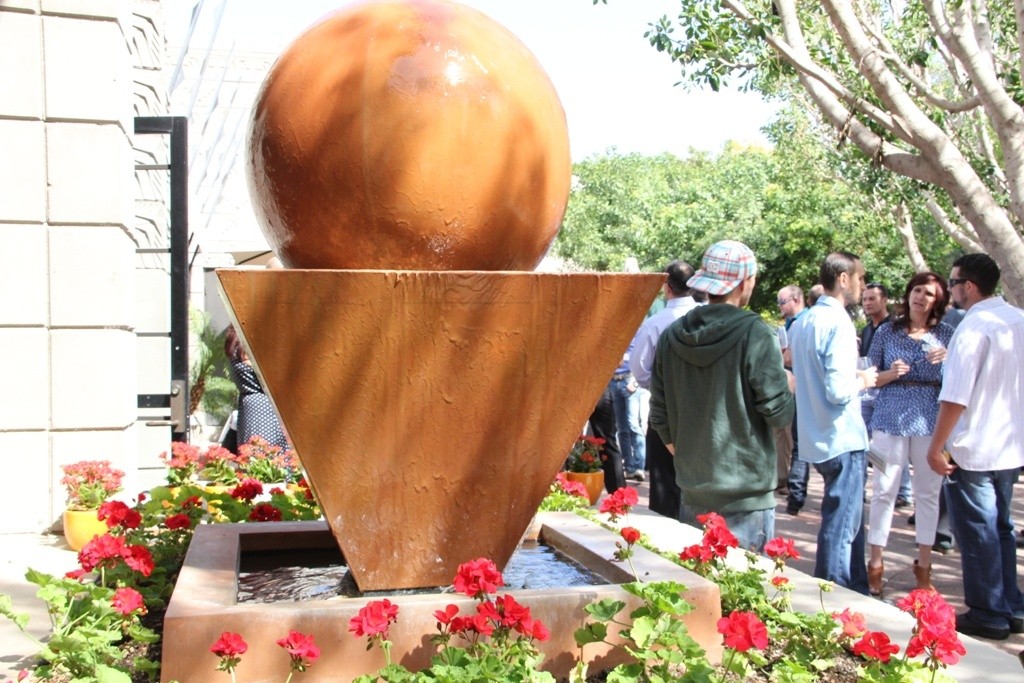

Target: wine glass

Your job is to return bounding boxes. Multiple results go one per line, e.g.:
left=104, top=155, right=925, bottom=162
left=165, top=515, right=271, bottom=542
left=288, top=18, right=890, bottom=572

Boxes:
left=855, top=354, right=877, bottom=401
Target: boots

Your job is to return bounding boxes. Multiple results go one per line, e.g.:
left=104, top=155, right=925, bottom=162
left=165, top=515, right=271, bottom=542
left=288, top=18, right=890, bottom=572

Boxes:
left=912, top=559, right=936, bottom=592
left=867, top=560, right=883, bottom=595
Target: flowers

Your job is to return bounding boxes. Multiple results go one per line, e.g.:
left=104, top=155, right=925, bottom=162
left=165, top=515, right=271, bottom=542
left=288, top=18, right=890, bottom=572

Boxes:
left=62, top=459, right=124, bottom=510
left=570, top=433, right=607, bottom=473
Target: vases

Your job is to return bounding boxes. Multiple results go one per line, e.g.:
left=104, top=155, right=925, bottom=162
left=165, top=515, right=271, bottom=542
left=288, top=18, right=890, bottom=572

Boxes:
left=567, top=468, right=605, bottom=505
left=63, top=505, right=110, bottom=553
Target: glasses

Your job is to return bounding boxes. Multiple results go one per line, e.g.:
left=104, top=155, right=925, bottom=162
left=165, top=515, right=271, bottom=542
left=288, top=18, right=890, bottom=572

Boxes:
left=777, top=297, right=793, bottom=304
left=948, top=278, right=967, bottom=288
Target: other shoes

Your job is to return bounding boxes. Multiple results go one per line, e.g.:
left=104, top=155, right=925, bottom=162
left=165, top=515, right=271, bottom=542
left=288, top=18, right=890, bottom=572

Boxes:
left=775, top=485, right=1024, bottom=556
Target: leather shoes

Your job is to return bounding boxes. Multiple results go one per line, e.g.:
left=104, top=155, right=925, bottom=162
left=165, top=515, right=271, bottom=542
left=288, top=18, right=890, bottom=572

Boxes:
left=956, top=612, right=1010, bottom=640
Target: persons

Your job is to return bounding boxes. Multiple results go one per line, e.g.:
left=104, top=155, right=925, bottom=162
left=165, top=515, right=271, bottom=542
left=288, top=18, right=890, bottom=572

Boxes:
left=648, top=239, right=796, bottom=561
left=606, top=329, right=648, bottom=482
left=630, top=261, right=707, bottom=523
left=789, top=251, right=878, bottom=602
left=587, top=385, right=626, bottom=497
left=222, top=323, right=298, bottom=472
left=767, top=275, right=1024, bottom=555
left=926, top=252, right=1024, bottom=643
left=862, top=271, right=960, bottom=605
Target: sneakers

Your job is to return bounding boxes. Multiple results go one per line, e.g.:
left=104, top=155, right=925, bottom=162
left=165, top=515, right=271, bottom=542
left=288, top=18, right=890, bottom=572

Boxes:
left=624, top=469, right=646, bottom=481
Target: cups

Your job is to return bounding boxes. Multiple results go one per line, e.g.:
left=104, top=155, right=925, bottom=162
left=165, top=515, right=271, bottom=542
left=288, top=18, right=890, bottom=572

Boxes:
left=772, top=324, right=788, bottom=356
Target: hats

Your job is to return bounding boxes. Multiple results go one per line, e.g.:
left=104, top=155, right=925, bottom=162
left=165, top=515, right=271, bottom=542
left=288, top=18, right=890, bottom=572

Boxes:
left=686, top=239, right=757, bottom=295
